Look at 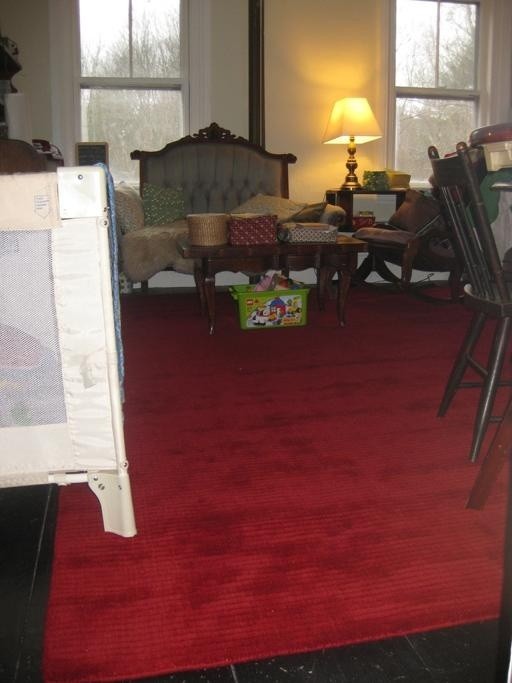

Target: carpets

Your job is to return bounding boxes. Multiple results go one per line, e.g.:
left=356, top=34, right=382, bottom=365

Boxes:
left=41, top=281, right=512, bottom=683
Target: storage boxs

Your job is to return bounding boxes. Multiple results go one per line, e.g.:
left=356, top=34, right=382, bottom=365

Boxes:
left=228, top=284, right=312, bottom=330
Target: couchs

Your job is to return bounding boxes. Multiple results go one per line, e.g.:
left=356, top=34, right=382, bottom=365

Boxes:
left=120, top=124, right=347, bottom=300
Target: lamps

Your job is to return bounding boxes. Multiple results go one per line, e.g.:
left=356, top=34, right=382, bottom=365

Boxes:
left=321, top=97, right=382, bottom=190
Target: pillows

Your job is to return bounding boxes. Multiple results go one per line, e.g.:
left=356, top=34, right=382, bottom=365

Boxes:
left=291, top=202, right=327, bottom=220
left=115, top=180, right=144, bottom=235
left=143, top=182, right=186, bottom=225
left=388, top=188, right=443, bottom=233
left=229, top=193, right=307, bottom=224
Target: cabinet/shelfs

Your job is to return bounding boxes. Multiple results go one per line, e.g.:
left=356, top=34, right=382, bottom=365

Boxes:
left=325, top=189, right=471, bottom=282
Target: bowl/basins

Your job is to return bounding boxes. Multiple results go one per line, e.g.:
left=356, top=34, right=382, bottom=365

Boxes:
left=351, top=215, right=376, bottom=229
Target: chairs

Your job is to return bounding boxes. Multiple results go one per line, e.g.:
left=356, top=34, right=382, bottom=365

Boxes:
left=349, top=172, right=470, bottom=303
left=428, top=142, right=512, bottom=464
